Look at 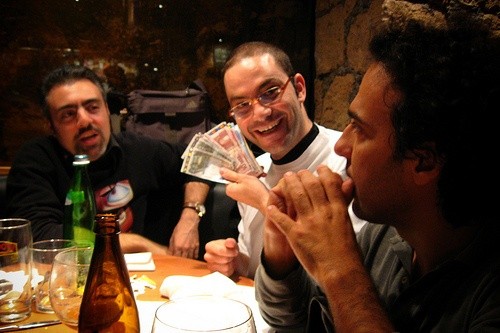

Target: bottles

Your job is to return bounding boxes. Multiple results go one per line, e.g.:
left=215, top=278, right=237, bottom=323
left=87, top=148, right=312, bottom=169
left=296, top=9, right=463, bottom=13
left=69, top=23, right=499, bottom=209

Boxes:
left=64, top=154, right=95, bottom=249
left=78, top=214, right=140, bottom=333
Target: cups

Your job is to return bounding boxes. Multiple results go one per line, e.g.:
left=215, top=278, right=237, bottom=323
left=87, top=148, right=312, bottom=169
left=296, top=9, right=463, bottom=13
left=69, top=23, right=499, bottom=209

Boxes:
left=50, top=248, right=94, bottom=326
left=151, top=297, right=257, bottom=333
left=28, top=239, right=79, bottom=313
left=0, top=218, right=33, bottom=323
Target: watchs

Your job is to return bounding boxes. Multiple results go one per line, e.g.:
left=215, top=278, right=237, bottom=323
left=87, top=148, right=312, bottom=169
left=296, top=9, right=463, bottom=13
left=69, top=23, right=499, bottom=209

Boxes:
left=183, top=201, right=206, bottom=217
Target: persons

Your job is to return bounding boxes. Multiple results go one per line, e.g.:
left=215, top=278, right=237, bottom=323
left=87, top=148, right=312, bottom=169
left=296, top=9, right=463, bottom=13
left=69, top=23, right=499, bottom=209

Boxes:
left=5, top=64, right=216, bottom=259
left=103, top=65, right=132, bottom=114
left=254, top=0, right=500, bottom=333
left=204, top=42, right=368, bottom=281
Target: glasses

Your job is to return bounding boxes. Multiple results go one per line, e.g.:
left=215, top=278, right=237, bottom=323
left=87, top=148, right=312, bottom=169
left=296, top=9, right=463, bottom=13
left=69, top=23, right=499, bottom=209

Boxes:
left=229, top=75, right=295, bottom=118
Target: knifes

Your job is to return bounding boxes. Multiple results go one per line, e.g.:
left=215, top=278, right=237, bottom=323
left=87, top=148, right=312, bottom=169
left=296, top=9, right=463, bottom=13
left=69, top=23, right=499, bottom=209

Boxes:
left=0, top=320, right=62, bottom=332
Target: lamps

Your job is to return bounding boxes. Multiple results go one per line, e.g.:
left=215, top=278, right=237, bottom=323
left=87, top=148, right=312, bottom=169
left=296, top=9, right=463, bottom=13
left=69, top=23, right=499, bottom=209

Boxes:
left=204, top=27, right=235, bottom=78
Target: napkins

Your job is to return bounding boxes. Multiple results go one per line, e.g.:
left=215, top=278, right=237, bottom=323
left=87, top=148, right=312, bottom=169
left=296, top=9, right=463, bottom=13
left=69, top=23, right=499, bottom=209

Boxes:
left=122, top=252, right=156, bottom=272
left=0, top=269, right=44, bottom=293
left=161, top=272, right=247, bottom=303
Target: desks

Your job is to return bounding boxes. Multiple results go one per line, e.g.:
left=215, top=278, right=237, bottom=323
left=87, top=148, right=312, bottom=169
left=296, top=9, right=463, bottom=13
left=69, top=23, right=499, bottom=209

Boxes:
left=0, top=252, right=274, bottom=333
